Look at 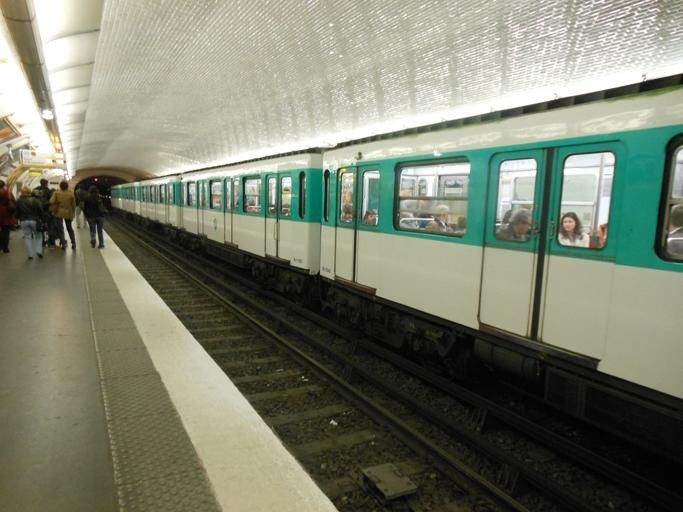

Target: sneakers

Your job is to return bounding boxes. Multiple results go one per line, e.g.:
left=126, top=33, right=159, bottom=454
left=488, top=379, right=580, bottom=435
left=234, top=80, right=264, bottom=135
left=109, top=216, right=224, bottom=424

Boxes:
left=28, top=252, right=44, bottom=261
left=90, top=239, right=105, bottom=248
left=28, top=239, right=76, bottom=250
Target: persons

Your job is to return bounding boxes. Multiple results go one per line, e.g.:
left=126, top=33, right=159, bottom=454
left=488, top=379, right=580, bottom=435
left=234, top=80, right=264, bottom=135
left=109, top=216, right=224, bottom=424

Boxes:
left=342, top=204, right=353, bottom=224
left=558, top=212, right=590, bottom=249
left=0, top=180, right=16, bottom=254
left=16, top=186, right=44, bottom=260
left=495, top=209, right=531, bottom=243
left=363, top=210, right=376, bottom=225
left=75, top=184, right=88, bottom=229
left=665, top=204, right=683, bottom=259
left=30, top=179, right=52, bottom=246
left=84, top=185, right=109, bottom=249
left=47, top=181, right=77, bottom=250
left=591, top=222, right=607, bottom=249
left=399, top=197, right=465, bottom=234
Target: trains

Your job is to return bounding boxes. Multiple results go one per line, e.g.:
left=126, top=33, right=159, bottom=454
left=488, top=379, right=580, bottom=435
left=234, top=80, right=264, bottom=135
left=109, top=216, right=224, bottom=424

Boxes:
left=109, top=71, right=683, bottom=460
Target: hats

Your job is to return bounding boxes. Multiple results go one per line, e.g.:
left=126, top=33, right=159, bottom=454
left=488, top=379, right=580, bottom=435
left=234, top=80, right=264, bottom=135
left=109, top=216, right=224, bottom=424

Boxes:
left=21, top=185, right=31, bottom=194
left=507, top=208, right=532, bottom=224
left=432, top=205, right=451, bottom=216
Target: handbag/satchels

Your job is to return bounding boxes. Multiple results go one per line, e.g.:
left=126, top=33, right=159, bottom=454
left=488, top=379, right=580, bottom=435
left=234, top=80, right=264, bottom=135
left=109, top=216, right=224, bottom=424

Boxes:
left=36, top=217, right=50, bottom=232
